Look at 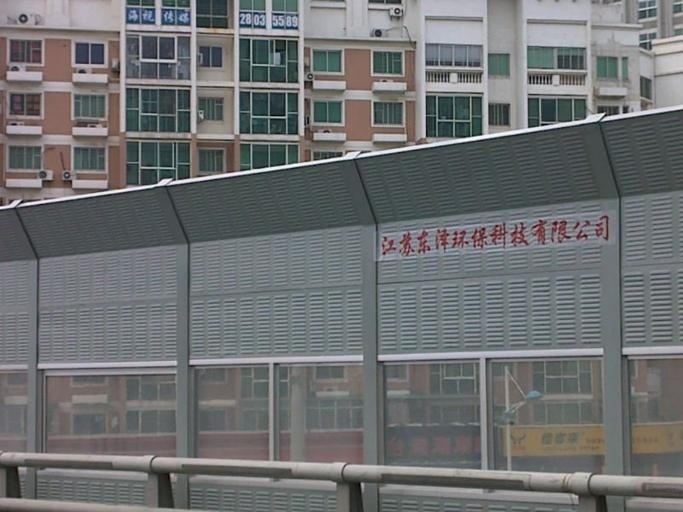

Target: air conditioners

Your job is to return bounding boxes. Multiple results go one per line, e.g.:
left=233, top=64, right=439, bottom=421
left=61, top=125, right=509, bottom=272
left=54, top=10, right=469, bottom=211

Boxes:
left=109, top=59, right=119, bottom=71
left=15, top=11, right=36, bottom=27
left=303, top=6, right=406, bottom=134
left=6, top=62, right=25, bottom=73
left=75, top=64, right=93, bottom=75
left=5, top=121, right=103, bottom=181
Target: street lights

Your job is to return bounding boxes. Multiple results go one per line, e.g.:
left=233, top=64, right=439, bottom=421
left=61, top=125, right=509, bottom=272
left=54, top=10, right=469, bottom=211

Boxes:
left=503, top=364, right=543, bottom=470
